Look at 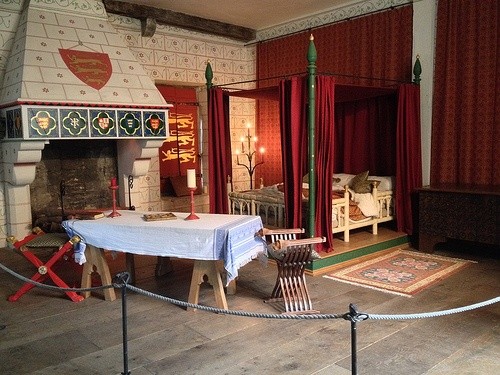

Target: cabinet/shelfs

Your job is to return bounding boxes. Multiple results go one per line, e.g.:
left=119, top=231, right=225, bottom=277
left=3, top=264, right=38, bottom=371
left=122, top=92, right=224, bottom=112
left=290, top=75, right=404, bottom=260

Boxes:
left=414, top=182, right=500, bottom=252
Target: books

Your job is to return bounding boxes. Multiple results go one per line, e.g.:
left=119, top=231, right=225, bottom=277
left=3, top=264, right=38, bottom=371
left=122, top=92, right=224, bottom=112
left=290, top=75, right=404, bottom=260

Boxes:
left=143, top=212, right=177, bottom=221
left=75, top=212, right=105, bottom=220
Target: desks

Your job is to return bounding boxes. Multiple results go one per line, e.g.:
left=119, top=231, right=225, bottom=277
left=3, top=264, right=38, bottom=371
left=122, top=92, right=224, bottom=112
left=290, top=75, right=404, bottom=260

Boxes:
left=62, top=211, right=269, bottom=312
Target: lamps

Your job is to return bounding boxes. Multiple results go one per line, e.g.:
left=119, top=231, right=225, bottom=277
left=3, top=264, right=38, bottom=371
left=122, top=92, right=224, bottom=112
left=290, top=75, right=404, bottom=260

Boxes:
left=236, top=122, right=265, bottom=189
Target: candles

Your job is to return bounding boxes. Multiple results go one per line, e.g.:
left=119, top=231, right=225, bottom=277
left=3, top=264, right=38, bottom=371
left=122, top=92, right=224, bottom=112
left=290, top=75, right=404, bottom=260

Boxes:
left=187, top=168, right=196, bottom=188
left=111, top=177, right=117, bottom=186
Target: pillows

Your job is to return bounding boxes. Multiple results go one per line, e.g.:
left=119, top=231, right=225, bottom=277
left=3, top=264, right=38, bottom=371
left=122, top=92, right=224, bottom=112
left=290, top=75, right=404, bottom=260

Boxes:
left=359, top=180, right=381, bottom=193
left=348, top=170, right=369, bottom=192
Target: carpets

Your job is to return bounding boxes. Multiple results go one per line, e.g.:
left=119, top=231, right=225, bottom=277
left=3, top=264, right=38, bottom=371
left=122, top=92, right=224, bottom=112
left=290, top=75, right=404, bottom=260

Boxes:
left=322, top=249, right=479, bottom=298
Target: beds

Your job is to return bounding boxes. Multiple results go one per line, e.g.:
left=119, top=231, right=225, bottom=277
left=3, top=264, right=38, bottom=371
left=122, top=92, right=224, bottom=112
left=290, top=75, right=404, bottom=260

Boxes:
left=227, top=169, right=398, bottom=243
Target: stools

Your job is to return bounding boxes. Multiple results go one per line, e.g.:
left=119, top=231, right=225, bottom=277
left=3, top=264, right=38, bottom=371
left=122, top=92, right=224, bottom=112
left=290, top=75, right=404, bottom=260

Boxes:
left=7, top=227, right=84, bottom=303
left=259, top=226, right=328, bottom=317
